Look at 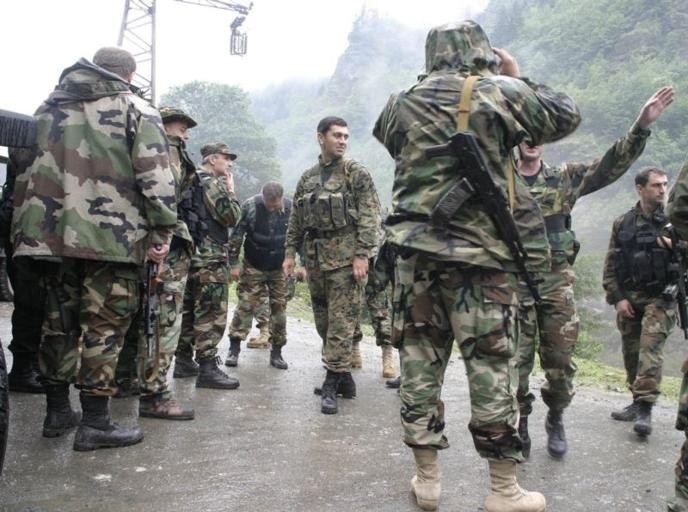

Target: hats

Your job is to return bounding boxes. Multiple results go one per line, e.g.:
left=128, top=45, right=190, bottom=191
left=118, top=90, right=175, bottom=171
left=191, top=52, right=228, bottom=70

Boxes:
left=201, top=142, right=237, bottom=160
left=425, top=20, right=502, bottom=72
left=160, top=106, right=197, bottom=128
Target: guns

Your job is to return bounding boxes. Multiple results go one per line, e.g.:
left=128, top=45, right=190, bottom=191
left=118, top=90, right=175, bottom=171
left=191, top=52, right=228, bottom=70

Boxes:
left=426, top=131, right=547, bottom=305
left=661, top=223, right=688, bottom=335
left=142, top=243, right=162, bottom=359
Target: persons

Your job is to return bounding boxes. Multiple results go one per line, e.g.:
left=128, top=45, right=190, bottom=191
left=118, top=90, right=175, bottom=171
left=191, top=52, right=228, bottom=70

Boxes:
left=603, top=167, right=678, bottom=435
left=111, top=105, right=202, bottom=421
left=281, top=117, right=377, bottom=415
left=350, top=210, right=396, bottom=378
left=225, top=181, right=293, bottom=369
left=248, top=273, right=295, bottom=350
left=8, top=47, right=178, bottom=452
left=0, top=145, right=48, bottom=393
left=667, top=161, right=688, bottom=502
left=172, top=141, right=241, bottom=389
left=372, top=19, right=580, bottom=510
left=512, top=85, right=675, bottom=460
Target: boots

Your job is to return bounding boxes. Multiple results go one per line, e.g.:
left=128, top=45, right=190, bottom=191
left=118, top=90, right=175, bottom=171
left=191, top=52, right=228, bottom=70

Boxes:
left=485, top=458, right=546, bottom=512
left=226, top=328, right=400, bottom=415
left=411, top=449, right=441, bottom=511
left=546, top=407, right=568, bottom=458
left=519, top=416, right=530, bottom=448
left=611, top=401, right=639, bottom=421
left=8, top=355, right=240, bottom=451
left=634, top=403, right=653, bottom=436
